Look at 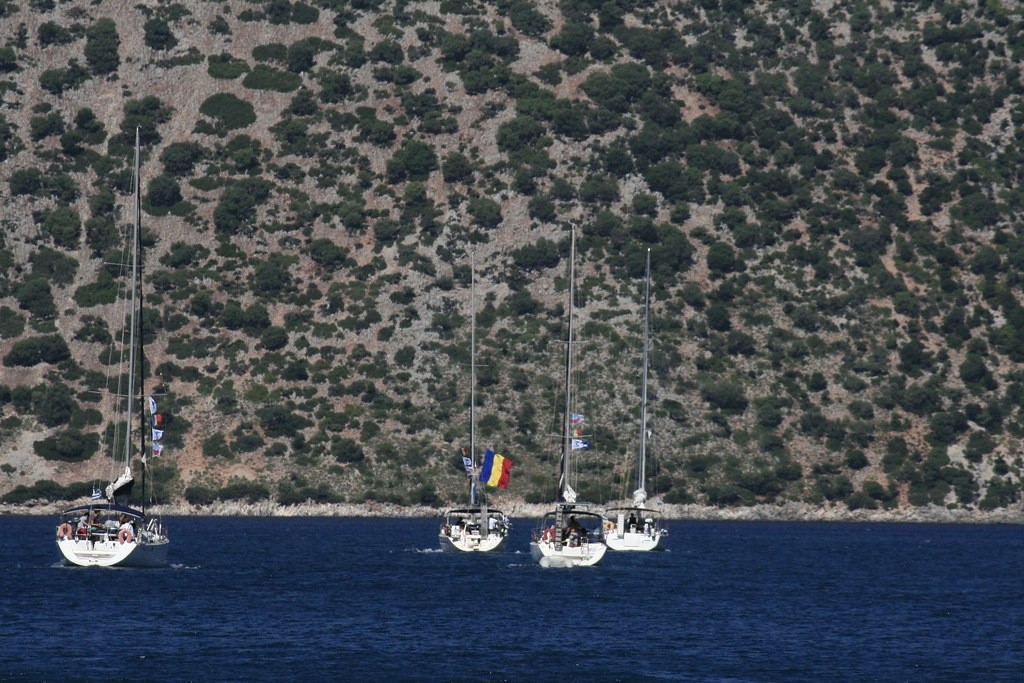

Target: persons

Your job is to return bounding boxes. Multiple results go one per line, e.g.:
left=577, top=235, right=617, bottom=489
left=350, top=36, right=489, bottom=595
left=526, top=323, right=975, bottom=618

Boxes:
left=75, top=510, right=102, bottom=540
left=455, top=517, right=465, bottom=529
left=628, top=513, right=636, bottom=529
left=117, top=513, right=138, bottom=541
left=565, top=516, right=581, bottom=547
left=488, top=515, right=498, bottom=534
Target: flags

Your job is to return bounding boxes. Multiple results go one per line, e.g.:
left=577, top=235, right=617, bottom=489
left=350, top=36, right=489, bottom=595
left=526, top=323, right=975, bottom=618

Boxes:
left=572, top=414, right=585, bottom=438
left=571, top=438, right=590, bottom=450
left=149, top=396, right=164, bottom=457
left=479, top=449, right=513, bottom=489
left=462, top=455, right=477, bottom=473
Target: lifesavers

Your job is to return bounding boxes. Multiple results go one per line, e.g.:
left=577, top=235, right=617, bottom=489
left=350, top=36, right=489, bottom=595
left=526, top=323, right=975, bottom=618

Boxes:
left=58, top=523, right=73, bottom=538
left=119, top=529, right=133, bottom=545
left=605, top=522, right=614, bottom=531
left=543, top=528, right=556, bottom=544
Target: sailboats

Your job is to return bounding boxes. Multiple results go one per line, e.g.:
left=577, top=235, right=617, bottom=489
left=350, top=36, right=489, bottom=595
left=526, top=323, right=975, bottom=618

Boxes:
left=55, top=123, right=171, bottom=569
left=438, top=249, right=514, bottom=553
left=529, top=226, right=609, bottom=569
left=600, top=246, right=668, bottom=553
